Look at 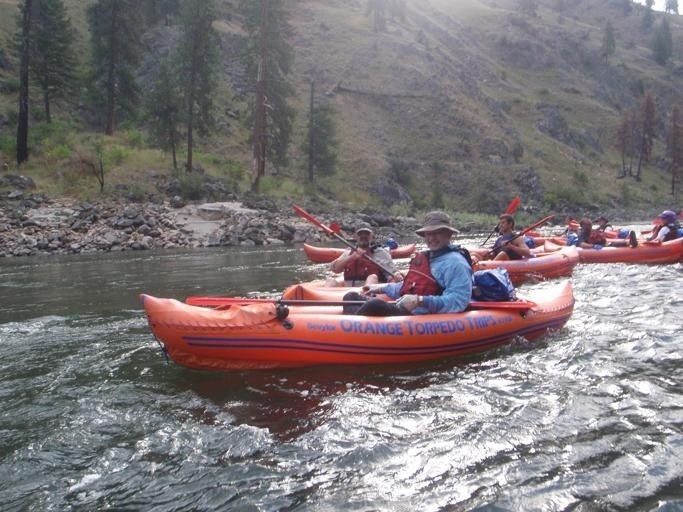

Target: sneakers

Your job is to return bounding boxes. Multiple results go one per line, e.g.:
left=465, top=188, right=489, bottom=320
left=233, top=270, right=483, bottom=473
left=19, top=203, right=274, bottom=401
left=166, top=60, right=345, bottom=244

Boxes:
left=629, top=231, right=637, bottom=247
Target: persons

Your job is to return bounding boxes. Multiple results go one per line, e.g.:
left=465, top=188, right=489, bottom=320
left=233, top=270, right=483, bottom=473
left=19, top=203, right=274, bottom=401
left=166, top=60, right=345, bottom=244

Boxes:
left=330, top=221, right=404, bottom=286
left=487, top=213, right=531, bottom=261
left=556, top=211, right=683, bottom=251
left=342, top=209, right=473, bottom=316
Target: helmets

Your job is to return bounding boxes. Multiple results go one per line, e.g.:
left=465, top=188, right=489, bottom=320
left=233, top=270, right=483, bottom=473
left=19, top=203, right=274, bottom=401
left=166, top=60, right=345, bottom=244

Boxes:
left=659, top=210, right=677, bottom=221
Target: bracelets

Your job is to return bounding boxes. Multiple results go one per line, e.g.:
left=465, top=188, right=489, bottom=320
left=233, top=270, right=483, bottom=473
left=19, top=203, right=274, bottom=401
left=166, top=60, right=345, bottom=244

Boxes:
left=418, top=294, right=425, bottom=307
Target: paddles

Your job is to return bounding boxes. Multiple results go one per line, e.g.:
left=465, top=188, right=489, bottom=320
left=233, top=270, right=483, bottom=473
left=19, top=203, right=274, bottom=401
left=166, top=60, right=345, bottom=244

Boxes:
left=184, top=296, right=536, bottom=309
left=471, top=214, right=555, bottom=265
left=640, top=231, right=652, bottom=234
left=478, top=196, right=520, bottom=248
left=329, top=223, right=348, bottom=243
left=290, top=203, right=394, bottom=279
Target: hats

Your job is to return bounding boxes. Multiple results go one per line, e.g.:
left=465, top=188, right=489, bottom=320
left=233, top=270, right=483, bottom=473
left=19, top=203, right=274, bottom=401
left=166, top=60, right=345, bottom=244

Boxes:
left=356, top=222, right=372, bottom=234
left=415, top=211, right=460, bottom=237
left=597, top=216, right=608, bottom=223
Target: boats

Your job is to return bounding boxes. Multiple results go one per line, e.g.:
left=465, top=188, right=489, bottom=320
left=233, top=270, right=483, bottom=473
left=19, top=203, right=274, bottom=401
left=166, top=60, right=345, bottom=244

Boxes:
left=136, top=276, right=577, bottom=367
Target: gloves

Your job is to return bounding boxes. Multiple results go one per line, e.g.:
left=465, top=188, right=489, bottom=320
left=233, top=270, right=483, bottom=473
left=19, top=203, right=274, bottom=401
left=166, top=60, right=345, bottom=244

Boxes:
left=362, top=283, right=383, bottom=295
left=592, top=244, right=602, bottom=249
left=395, top=294, right=420, bottom=311
